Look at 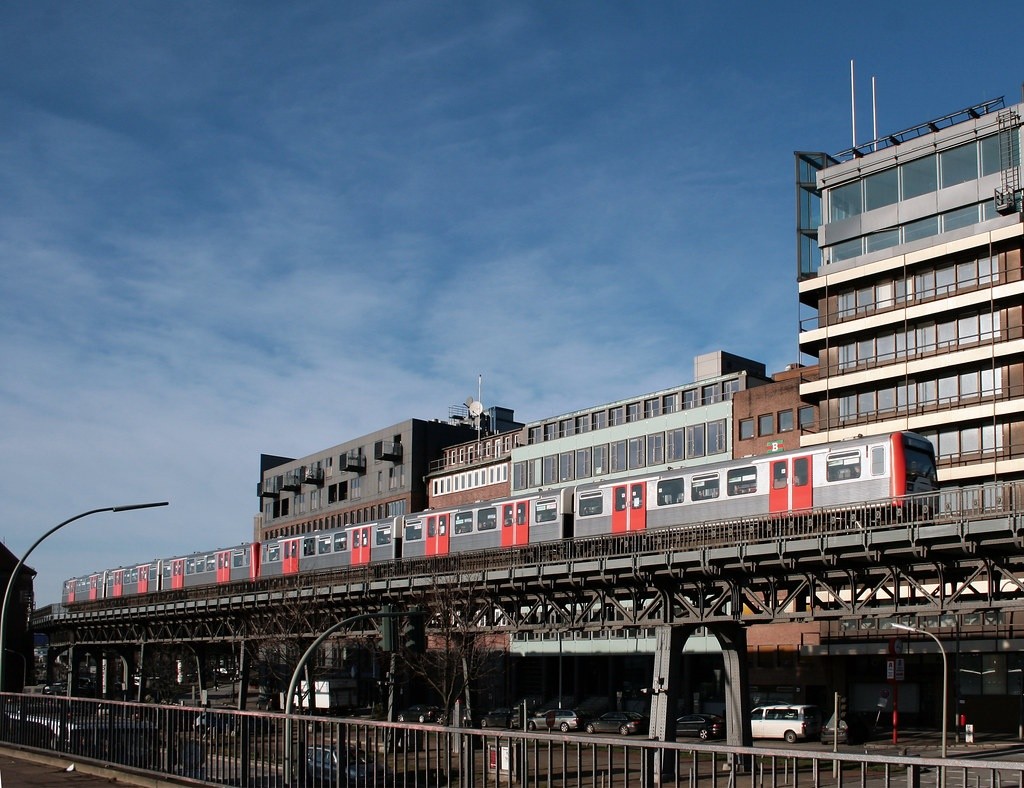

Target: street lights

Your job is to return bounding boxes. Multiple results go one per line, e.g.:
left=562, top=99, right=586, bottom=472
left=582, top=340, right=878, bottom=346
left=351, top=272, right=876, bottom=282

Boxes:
left=1, top=501, right=173, bottom=641
left=887, top=621, right=948, bottom=787
left=284, top=608, right=426, bottom=788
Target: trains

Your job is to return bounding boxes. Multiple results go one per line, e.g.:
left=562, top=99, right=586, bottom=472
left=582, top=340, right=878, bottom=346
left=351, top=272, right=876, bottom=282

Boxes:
left=60, top=429, right=960, bottom=610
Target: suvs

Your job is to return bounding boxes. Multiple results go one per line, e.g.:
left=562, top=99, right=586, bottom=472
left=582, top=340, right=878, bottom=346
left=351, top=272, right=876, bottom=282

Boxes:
left=305, top=743, right=391, bottom=787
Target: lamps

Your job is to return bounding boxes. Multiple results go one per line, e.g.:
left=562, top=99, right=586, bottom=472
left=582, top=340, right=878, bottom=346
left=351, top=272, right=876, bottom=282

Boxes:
left=640, top=687, right=665, bottom=697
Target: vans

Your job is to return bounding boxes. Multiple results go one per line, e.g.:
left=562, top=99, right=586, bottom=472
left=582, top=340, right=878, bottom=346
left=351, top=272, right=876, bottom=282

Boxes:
left=751, top=703, right=820, bottom=744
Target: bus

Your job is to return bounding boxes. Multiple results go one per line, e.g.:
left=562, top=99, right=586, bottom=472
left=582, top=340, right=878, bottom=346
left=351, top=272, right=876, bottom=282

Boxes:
left=6, top=706, right=158, bottom=765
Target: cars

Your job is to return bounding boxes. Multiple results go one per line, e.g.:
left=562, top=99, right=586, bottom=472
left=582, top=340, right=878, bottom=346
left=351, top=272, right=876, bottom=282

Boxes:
left=821, top=709, right=871, bottom=746
left=396, top=704, right=440, bottom=723
left=527, top=709, right=582, bottom=733
left=191, top=705, right=262, bottom=733
left=437, top=705, right=521, bottom=729
left=675, top=713, right=726, bottom=742
left=583, top=711, right=649, bottom=736
